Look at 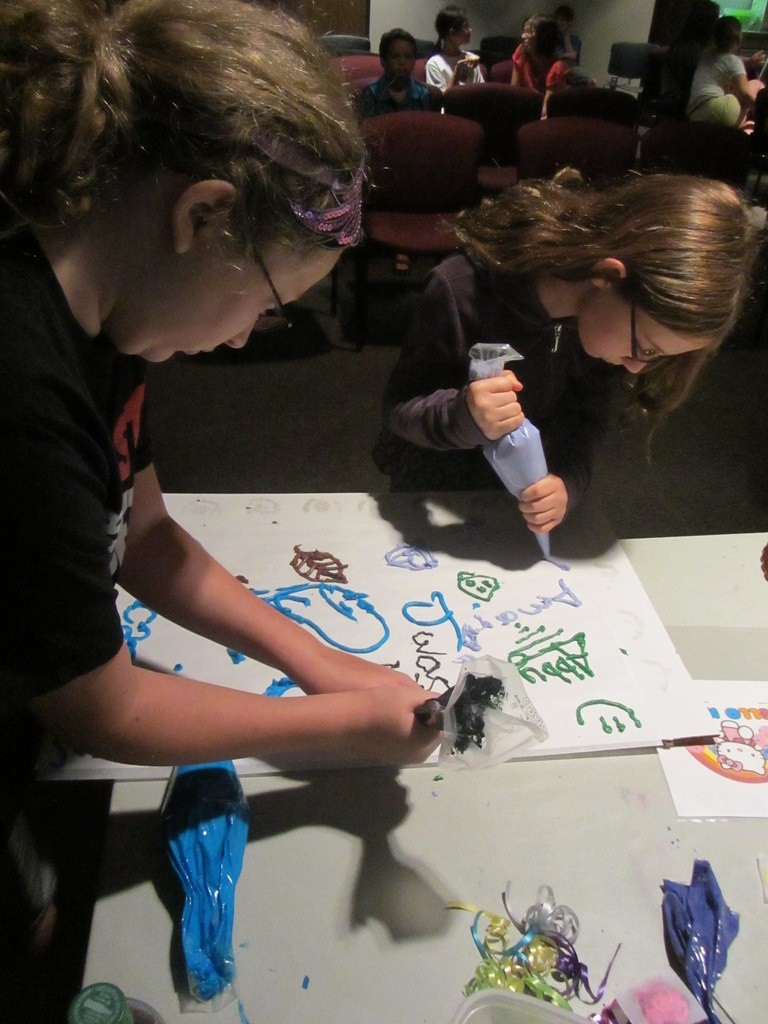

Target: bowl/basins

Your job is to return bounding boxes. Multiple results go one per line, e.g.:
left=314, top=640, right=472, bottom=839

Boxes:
left=449, top=987, right=597, bottom=1024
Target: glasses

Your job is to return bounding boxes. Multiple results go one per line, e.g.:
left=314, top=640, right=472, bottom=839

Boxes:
left=617, top=298, right=664, bottom=364
left=195, top=167, right=293, bottom=334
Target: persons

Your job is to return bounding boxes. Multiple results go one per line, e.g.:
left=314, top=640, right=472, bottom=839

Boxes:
left=425, top=7, right=484, bottom=94
left=687, top=15, right=765, bottom=129
left=650, top=0, right=720, bottom=115
left=510, top=16, right=568, bottom=118
left=372, top=172, right=759, bottom=531
left=553, top=5, right=580, bottom=66
left=350, top=28, right=444, bottom=123
left=747, top=64, right=768, bottom=171
left=0, top=0, right=446, bottom=1023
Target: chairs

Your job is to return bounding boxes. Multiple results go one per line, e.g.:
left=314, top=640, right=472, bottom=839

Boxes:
left=320, top=34, right=661, bottom=314
